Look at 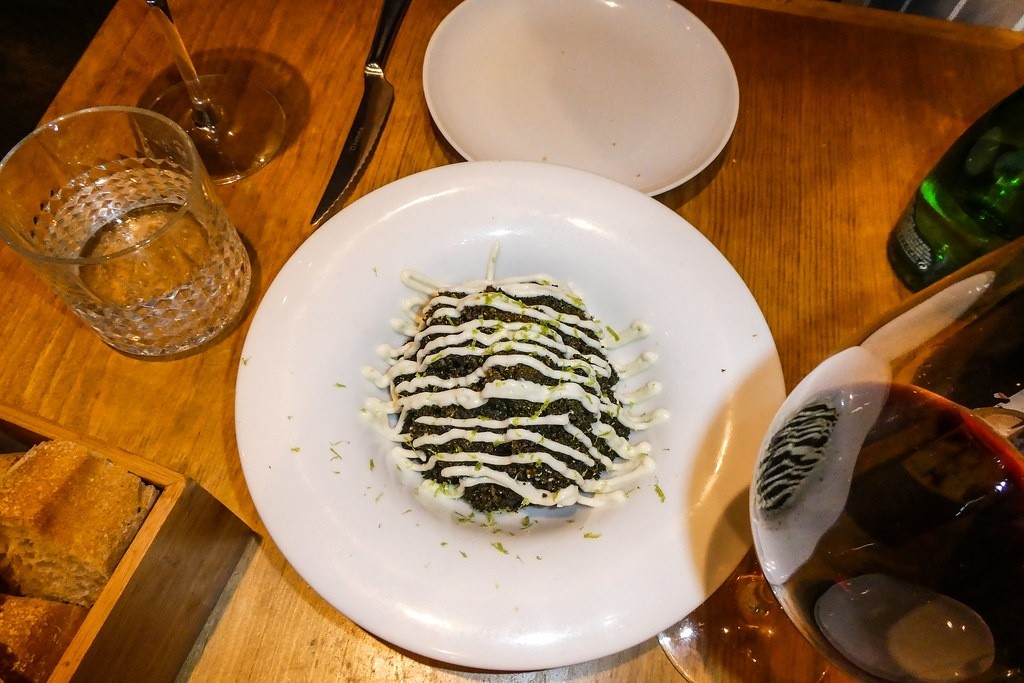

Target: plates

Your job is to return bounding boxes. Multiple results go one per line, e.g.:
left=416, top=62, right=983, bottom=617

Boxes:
left=423, top=0, right=738, bottom=195
left=235, top=162, right=788, bottom=670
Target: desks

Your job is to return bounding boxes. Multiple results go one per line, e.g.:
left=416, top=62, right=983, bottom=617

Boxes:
left=0, top=0, right=1024, bottom=683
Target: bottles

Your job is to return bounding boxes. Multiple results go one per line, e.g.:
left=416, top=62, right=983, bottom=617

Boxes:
left=886, top=83, right=1021, bottom=291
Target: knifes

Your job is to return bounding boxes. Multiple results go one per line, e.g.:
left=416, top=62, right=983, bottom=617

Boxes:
left=310, top=1, right=412, bottom=228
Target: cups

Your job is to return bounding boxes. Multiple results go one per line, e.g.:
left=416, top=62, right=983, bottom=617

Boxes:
left=2, top=107, right=252, bottom=356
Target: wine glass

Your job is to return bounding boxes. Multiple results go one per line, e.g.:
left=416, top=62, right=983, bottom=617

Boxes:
left=654, top=238, right=1021, bottom=681
left=125, top=1, right=285, bottom=185
